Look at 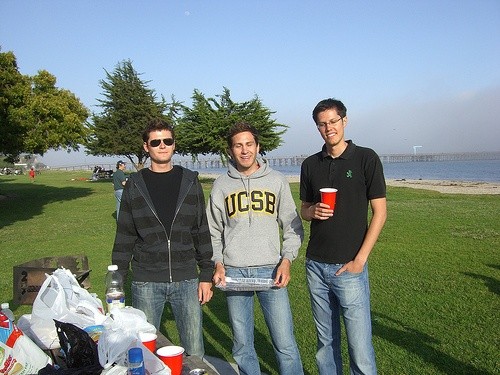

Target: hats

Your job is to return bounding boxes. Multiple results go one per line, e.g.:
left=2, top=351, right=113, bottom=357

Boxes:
left=116, top=160, right=127, bottom=166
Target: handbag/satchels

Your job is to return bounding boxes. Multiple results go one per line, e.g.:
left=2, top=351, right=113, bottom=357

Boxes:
left=29, top=268, right=157, bottom=375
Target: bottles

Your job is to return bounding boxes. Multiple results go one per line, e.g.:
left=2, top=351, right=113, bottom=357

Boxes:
left=126, top=347, right=146, bottom=375
left=105, top=264, right=126, bottom=318
left=1, top=302, right=16, bottom=325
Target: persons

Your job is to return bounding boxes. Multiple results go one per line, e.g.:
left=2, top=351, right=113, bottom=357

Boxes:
left=300, top=98, right=388, bottom=375
left=206, top=123, right=304, bottom=375
left=93, top=166, right=99, bottom=173
left=112, top=120, right=215, bottom=357
left=112, top=161, right=126, bottom=220
left=29, top=168, right=34, bottom=183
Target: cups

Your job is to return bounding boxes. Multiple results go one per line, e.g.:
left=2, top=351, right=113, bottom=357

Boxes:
left=319, top=187, right=338, bottom=214
left=156, top=345, right=185, bottom=375
left=140, top=332, right=158, bottom=355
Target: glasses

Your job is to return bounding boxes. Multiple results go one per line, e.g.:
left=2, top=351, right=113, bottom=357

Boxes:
left=149, top=138, right=175, bottom=147
left=316, top=117, right=342, bottom=129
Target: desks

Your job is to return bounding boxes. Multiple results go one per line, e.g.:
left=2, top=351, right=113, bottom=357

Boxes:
left=44, top=330, right=218, bottom=375
